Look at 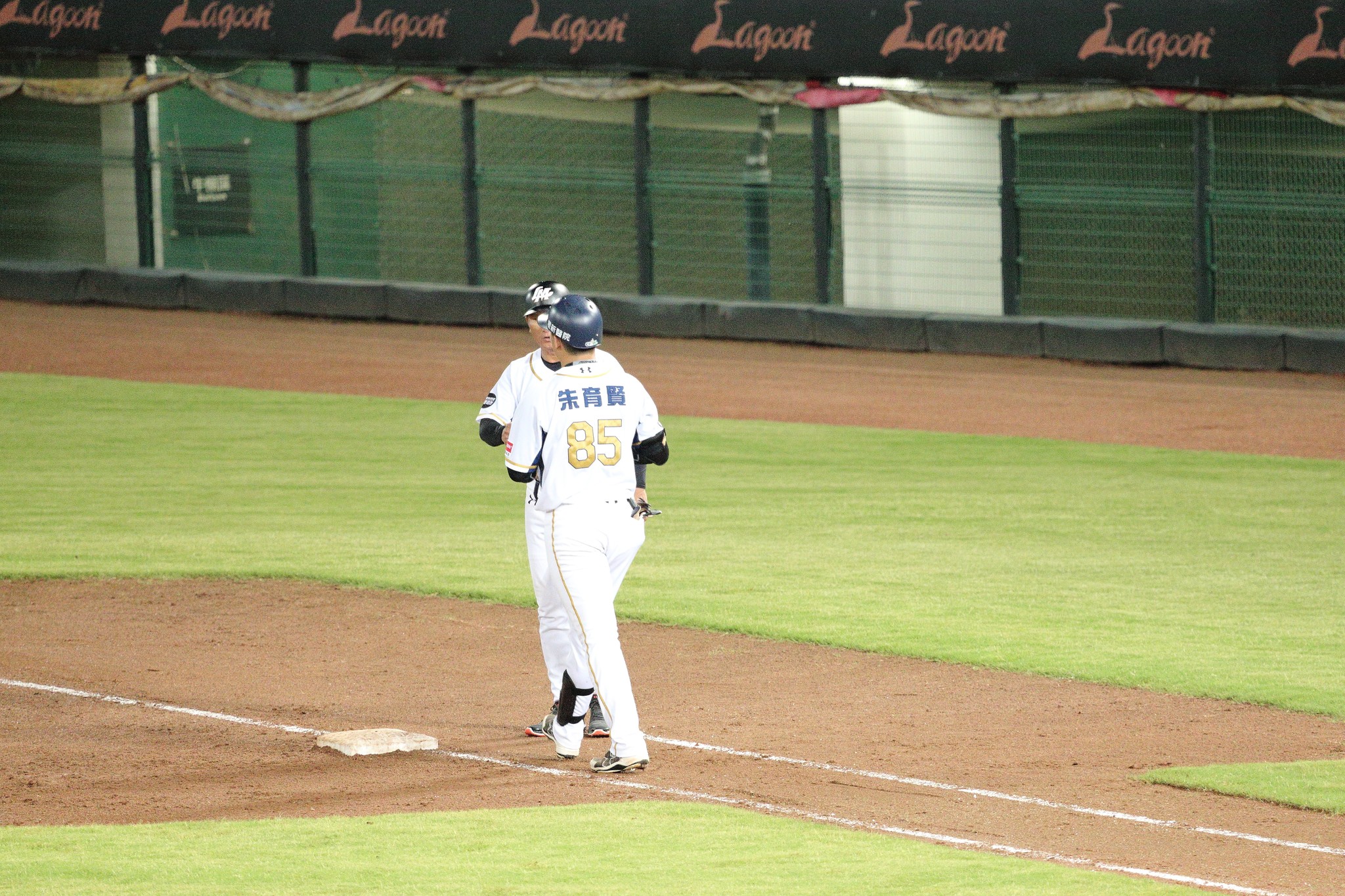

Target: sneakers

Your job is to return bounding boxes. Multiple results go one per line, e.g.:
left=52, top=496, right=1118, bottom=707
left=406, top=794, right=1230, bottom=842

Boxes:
left=542, top=715, right=575, bottom=760
left=583, top=694, right=611, bottom=737
left=589, top=751, right=650, bottom=774
left=525, top=700, right=587, bottom=737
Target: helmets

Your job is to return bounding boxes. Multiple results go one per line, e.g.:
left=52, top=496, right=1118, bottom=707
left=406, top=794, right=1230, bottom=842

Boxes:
left=537, top=295, right=603, bottom=350
left=523, top=282, right=570, bottom=316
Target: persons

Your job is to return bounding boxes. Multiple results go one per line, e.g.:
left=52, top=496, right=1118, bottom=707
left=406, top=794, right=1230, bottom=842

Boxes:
left=504, top=294, right=669, bottom=773
left=474, top=281, right=649, bottom=739
